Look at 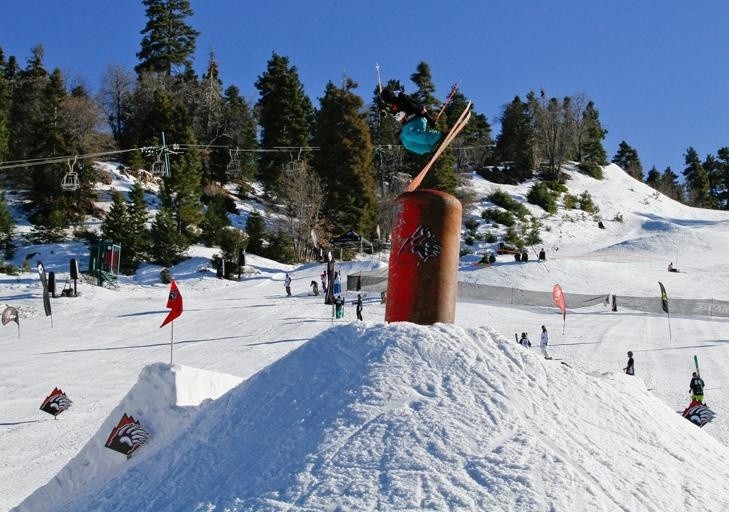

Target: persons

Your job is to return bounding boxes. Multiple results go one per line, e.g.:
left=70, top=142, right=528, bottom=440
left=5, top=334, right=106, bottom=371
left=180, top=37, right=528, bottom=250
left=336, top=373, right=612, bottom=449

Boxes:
left=519, top=333, right=531, bottom=348
left=623, top=351, right=635, bottom=375
left=352, top=294, right=363, bottom=320
left=310, top=271, right=341, bottom=296
left=689, top=371, right=704, bottom=403
left=541, top=325, right=550, bottom=358
left=334, top=296, right=344, bottom=319
left=479, top=248, right=545, bottom=264
left=284, top=272, right=291, bottom=297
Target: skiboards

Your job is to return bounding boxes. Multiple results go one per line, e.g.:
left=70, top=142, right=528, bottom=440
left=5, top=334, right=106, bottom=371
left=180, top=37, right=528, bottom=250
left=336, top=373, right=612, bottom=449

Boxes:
left=406, top=100, right=471, bottom=192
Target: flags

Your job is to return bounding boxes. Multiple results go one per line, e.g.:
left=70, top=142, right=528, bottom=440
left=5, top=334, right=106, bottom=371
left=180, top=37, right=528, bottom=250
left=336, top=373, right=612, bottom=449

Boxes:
left=36, top=260, right=52, bottom=317
left=161, top=281, right=182, bottom=328
left=553, top=284, right=565, bottom=320
left=658, top=282, right=668, bottom=314
